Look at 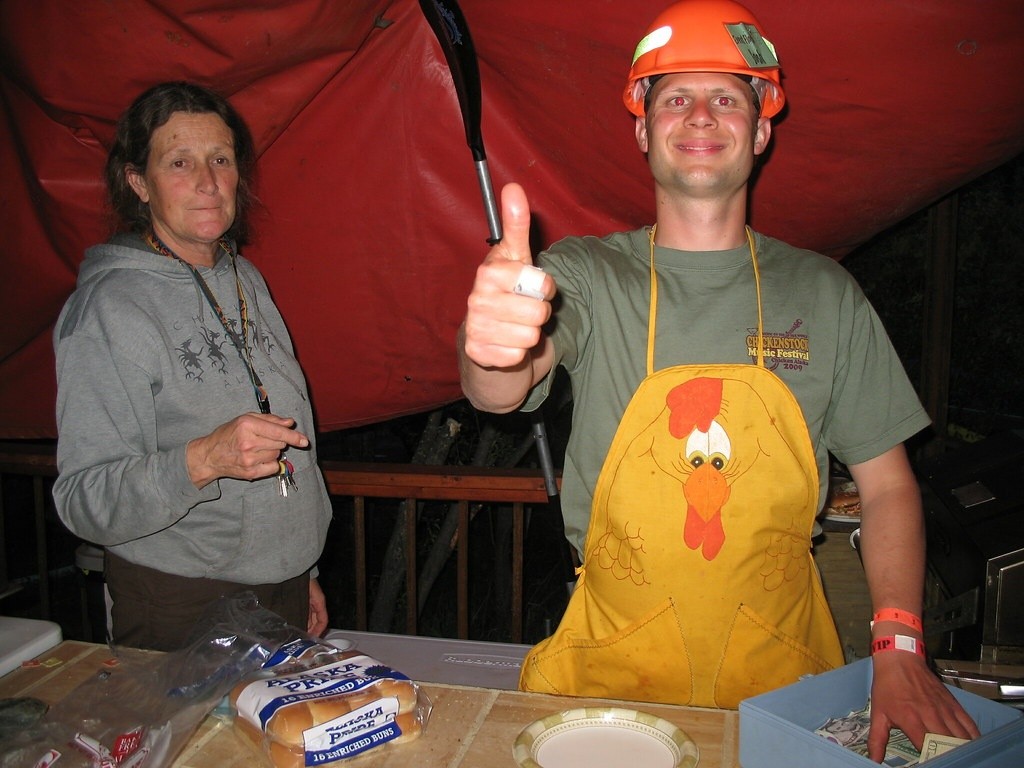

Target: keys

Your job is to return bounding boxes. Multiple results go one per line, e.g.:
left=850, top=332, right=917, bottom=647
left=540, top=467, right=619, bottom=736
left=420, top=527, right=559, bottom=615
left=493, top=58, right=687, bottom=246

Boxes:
left=274, top=461, right=298, bottom=498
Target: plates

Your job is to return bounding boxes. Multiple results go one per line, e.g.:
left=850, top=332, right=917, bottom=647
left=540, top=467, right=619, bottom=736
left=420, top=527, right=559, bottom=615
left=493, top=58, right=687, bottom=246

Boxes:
left=513, top=708, right=700, bottom=768
left=818, top=514, right=861, bottom=522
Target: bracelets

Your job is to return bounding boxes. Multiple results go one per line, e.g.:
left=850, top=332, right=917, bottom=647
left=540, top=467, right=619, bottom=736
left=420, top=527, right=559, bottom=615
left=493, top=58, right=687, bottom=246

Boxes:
left=870, top=608, right=923, bottom=636
left=871, top=632, right=925, bottom=657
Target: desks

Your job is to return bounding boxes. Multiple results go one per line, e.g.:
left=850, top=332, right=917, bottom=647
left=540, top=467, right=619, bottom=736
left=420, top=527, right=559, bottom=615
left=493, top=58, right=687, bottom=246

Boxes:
left=0, top=639, right=739, bottom=768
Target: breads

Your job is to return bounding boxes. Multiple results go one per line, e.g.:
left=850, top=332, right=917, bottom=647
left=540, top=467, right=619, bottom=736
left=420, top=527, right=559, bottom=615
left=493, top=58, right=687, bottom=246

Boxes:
left=229, top=649, right=422, bottom=768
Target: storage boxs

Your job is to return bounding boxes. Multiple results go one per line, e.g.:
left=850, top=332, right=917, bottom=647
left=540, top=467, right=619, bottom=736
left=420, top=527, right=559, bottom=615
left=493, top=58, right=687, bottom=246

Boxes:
left=737, top=656, right=1024, bottom=768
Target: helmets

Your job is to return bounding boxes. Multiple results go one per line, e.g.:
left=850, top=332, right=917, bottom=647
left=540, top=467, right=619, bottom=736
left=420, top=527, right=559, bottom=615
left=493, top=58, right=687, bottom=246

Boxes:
left=623, top=0, right=786, bottom=118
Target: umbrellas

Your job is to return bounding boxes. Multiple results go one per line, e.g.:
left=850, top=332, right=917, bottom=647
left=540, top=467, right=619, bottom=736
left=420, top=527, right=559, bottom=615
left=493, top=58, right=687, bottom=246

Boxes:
left=0, top=0, right=1024, bottom=586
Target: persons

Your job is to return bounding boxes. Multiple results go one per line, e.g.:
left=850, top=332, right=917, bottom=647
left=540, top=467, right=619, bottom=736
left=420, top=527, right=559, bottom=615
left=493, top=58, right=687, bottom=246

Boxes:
left=456, top=0, right=982, bottom=762
left=51, top=80, right=333, bottom=655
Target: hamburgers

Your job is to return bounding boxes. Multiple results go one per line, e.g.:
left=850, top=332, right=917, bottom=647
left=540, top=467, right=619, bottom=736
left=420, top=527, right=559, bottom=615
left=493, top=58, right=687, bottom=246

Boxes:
left=821, top=494, right=861, bottom=515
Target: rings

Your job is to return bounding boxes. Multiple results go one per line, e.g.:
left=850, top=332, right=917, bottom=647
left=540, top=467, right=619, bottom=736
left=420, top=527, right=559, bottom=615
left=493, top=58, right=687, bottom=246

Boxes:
left=515, top=264, right=547, bottom=302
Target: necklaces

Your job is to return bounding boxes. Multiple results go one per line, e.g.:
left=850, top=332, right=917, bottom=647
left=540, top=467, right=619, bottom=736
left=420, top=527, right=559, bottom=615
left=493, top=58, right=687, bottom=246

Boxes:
left=649, top=225, right=655, bottom=245
